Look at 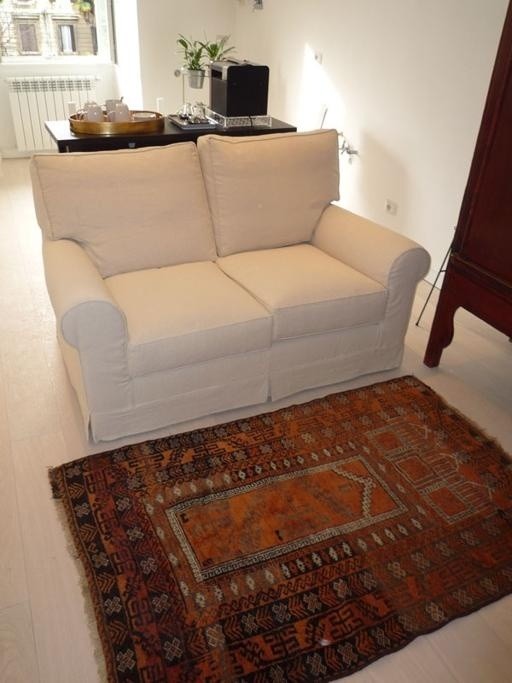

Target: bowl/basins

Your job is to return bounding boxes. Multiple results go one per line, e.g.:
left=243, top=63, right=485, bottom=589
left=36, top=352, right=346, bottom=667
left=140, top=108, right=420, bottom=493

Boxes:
left=132, top=112, right=156, bottom=122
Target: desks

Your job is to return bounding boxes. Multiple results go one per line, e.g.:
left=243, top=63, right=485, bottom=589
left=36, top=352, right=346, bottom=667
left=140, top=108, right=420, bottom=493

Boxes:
left=44, top=114, right=297, bottom=153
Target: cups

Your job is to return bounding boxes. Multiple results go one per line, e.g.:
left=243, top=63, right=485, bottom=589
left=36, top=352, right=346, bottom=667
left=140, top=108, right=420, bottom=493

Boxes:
left=76, top=99, right=129, bottom=122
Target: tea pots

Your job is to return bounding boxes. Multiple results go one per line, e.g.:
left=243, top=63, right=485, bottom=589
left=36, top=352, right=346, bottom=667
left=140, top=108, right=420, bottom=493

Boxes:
left=177, top=103, right=192, bottom=119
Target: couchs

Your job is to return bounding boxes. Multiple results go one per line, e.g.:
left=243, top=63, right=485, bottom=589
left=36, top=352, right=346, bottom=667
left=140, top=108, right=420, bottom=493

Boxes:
left=28, top=130, right=430, bottom=443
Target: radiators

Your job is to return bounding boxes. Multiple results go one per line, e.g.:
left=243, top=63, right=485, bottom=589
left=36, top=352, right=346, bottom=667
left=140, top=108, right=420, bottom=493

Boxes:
left=4, top=76, right=96, bottom=154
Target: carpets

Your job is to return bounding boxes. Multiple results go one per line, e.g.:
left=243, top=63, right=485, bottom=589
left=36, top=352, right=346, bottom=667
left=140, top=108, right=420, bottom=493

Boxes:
left=51, top=373, right=510, bottom=681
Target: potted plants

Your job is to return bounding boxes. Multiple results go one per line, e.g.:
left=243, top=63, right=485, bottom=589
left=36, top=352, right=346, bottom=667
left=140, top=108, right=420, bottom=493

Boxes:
left=177, top=34, right=206, bottom=90
